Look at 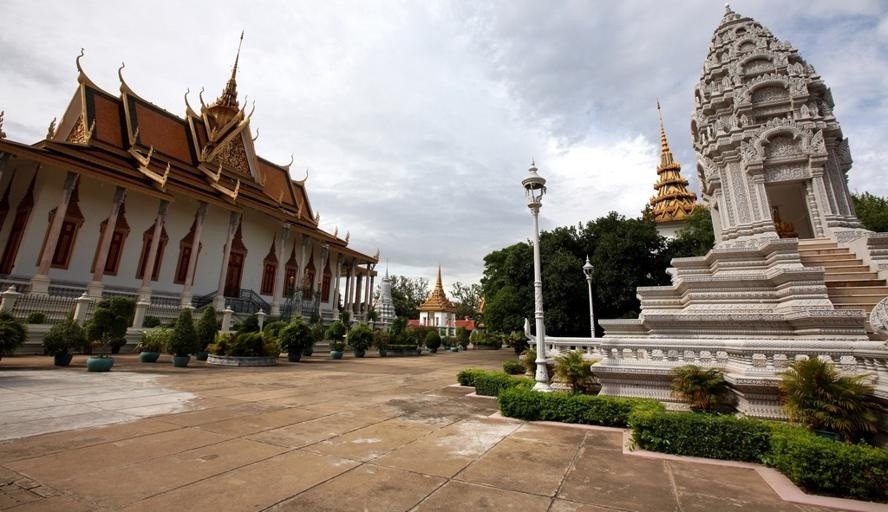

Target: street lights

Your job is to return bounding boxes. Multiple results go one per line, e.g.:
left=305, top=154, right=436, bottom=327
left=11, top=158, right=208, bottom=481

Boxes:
left=582, top=254, right=596, bottom=338
left=521, top=156, right=553, bottom=393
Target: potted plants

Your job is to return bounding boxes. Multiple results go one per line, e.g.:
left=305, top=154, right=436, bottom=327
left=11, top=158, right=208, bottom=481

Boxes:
left=524, top=348, right=603, bottom=396
left=194, top=305, right=218, bottom=361
left=132, top=325, right=169, bottom=363
left=263, top=314, right=503, bottom=362
left=780, top=356, right=884, bottom=445
left=43, top=318, right=87, bottom=367
left=671, top=362, right=740, bottom=415
left=166, top=307, right=199, bottom=368
left=84, top=307, right=127, bottom=372
